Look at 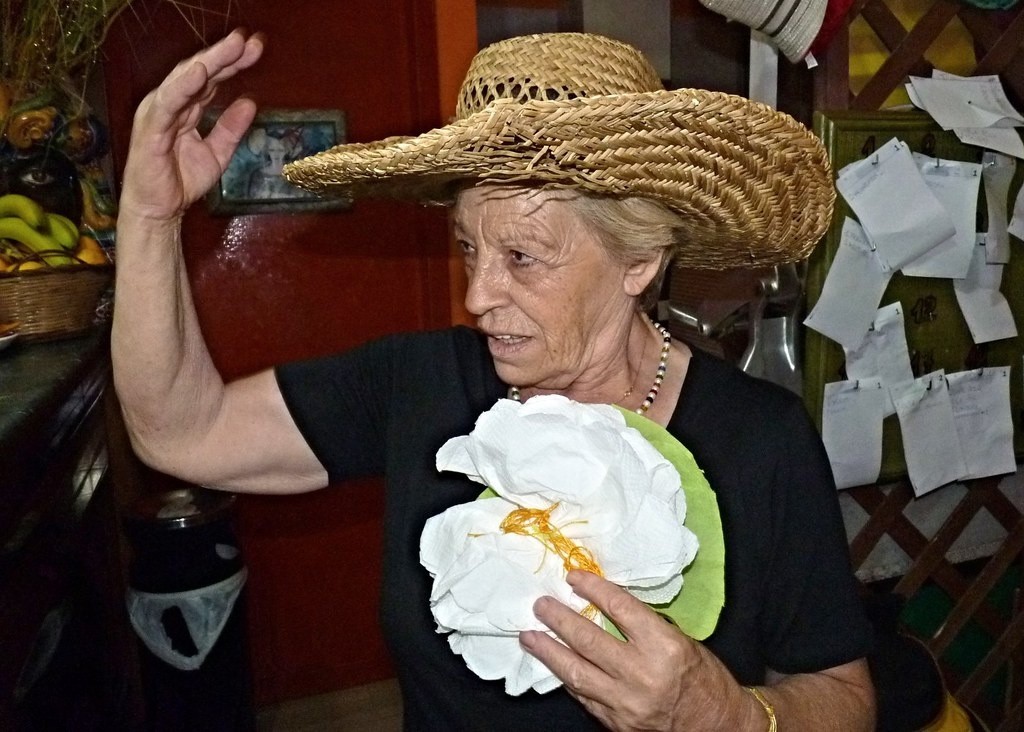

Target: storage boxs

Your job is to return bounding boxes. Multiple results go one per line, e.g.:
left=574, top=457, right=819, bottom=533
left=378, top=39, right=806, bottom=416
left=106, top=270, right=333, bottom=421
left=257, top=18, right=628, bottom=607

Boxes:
left=0, top=248, right=116, bottom=346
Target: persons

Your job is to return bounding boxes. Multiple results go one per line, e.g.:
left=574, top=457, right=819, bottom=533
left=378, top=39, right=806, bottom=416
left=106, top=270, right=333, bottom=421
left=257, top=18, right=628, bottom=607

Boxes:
left=106, top=27, right=878, bottom=732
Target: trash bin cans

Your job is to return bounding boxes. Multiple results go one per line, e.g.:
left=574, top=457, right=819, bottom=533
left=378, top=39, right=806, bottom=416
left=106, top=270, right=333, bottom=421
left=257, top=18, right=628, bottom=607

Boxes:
left=115, top=486, right=258, bottom=732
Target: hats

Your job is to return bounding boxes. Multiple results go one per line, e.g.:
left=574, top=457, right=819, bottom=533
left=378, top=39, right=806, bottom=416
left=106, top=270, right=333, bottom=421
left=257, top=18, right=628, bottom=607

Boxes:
left=281, top=32, right=832, bottom=273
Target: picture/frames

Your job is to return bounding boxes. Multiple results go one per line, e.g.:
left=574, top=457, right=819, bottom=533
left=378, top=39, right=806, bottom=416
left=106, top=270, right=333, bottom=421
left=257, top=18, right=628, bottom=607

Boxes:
left=200, top=106, right=356, bottom=218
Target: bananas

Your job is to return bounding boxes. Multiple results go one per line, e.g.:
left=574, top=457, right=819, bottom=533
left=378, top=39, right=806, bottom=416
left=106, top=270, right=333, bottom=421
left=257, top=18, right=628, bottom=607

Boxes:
left=1, top=192, right=109, bottom=277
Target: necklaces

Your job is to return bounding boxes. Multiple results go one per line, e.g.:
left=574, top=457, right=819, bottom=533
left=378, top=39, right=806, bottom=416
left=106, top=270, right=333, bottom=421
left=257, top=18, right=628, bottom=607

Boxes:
left=606, top=326, right=652, bottom=406
left=511, top=316, right=674, bottom=421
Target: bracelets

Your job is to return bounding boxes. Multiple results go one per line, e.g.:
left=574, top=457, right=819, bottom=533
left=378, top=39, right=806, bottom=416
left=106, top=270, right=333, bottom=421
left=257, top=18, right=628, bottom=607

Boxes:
left=745, top=685, right=779, bottom=732
